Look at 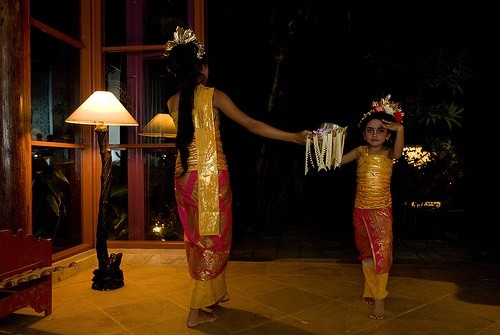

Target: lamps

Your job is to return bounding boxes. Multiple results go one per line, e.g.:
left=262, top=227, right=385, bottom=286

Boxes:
left=65, top=91, right=139, bottom=291
left=138, top=113, right=177, bottom=138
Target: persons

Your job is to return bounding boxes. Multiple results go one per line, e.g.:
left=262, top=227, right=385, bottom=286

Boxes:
left=319, top=94, right=405, bottom=321
left=162, top=26, right=323, bottom=327
